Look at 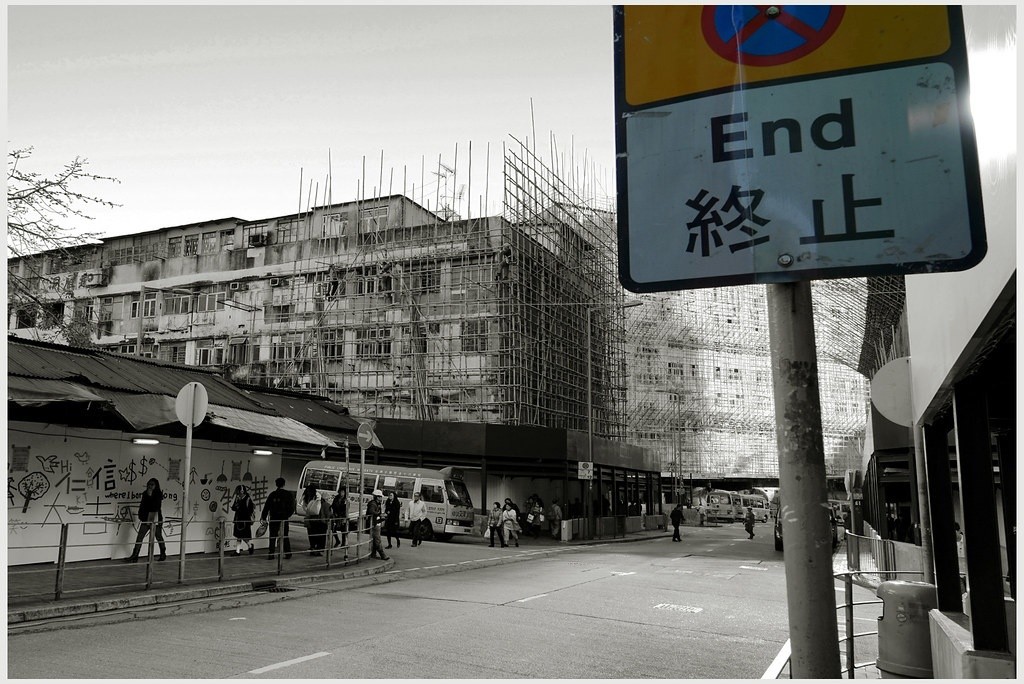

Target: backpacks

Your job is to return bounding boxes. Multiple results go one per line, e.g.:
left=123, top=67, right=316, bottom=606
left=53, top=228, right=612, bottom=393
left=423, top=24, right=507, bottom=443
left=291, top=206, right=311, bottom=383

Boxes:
left=306, top=492, right=322, bottom=516
left=545, top=504, right=559, bottom=521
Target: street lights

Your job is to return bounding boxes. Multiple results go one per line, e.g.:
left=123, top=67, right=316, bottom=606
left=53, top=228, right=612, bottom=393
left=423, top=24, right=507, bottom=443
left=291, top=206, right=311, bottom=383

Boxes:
left=653, top=388, right=683, bottom=505
left=584, top=299, right=643, bottom=541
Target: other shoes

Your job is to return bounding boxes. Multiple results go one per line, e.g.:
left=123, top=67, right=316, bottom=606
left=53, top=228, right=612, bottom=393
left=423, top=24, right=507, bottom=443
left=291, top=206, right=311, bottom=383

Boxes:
left=268, top=553, right=275, bottom=560
left=504, top=544, right=508, bottom=547
left=284, top=553, right=292, bottom=559
left=309, top=542, right=347, bottom=558
left=672, top=539, right=682, bottom=542
left=501, top=543, right=505, bottom=549
left=368, top=539, right=423, bottom=560
left=516, top=543, right=519, bottom=547
left=248, top=544, right=254, bottom=554
left=230, top=551, right=240, bottom=557
left=488, top=545, right=495, bottom=547
left=747, top=533, right=756, bottom=539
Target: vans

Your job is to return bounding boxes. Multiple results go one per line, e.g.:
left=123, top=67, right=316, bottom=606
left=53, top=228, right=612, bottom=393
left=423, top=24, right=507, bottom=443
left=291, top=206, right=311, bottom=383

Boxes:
left=731, top=493, right=775, bottom=522
left=705, top=492, right=739, bottom=523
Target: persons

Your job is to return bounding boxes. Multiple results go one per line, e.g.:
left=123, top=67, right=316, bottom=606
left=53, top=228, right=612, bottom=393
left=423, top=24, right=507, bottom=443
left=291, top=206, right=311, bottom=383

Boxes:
left=594, top=494, right=642, bottom=516
left=742, top=508, right=755, bottom=539
left=887, top=515, right=895, bottom=540
left=230, top=485, right=257, bottom=555
left=365, top=490, right=390, bottom=561
left=260, top=478, right=294, bottom=559
left=895, top=514, right=909, bottom=542
left=404, top=492, right=427, bottom=547
left=549, top=496, right=563, bottom=538
left=670, top=504, right=686, bottom=542
left=299, top=482, right=346, bottom=557
left=126, top=477, right=168, bottom=562
left=570, top=496, right=584, bottom=518
left=384, top=491, right=401, bottom=549
left=487, top=498, right=521, bottom=547
left=524, top=493, right=544, bottom=539
left=954, top=523, right=966, bottom=592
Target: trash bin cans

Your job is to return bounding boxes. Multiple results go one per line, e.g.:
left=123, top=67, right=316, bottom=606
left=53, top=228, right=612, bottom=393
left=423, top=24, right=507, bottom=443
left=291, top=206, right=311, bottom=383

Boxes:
left=875, top=579, right=937, bottom=678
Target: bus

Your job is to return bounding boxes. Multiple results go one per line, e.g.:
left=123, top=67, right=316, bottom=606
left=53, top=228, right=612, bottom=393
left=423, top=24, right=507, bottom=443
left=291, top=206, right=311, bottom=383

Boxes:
left=295, top=464, right=477, bottom=542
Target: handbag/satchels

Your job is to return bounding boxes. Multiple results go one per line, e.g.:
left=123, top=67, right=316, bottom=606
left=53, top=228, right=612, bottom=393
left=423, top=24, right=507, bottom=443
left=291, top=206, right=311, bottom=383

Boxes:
left=511, top=517, right=521, bottom=531
left=255, top=519, right=268, bottom=536
left=526, top=512, right=535, bottom=523
left=483, top=526, right=490, bottom=538
left=539, top=507, right=544, bottom=522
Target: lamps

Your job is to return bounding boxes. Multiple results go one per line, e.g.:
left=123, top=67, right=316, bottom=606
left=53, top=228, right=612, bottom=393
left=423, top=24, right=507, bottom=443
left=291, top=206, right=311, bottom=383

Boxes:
left=132, top=438, right=160, bottom=445
left=253, top=449, right=273, bottom=455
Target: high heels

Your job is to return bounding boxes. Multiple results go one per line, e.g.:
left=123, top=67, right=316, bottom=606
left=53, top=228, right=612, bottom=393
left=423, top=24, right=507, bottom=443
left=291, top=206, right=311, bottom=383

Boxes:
left=124, top=556, right=138, bottom=563
left=157, top=553, right=166, bottom=561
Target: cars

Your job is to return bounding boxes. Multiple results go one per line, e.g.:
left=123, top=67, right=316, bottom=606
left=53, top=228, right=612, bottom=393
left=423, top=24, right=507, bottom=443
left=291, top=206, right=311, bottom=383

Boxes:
left=769, top=498, right=849, bottom=552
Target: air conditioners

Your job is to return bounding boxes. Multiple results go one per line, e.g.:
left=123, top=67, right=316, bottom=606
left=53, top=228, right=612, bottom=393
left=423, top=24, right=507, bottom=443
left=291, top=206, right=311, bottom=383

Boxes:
left=86, top=268, right=110, bottom=286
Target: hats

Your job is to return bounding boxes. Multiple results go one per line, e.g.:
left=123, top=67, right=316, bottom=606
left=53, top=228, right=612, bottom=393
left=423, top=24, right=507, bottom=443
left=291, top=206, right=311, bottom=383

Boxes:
left=746, top=506, right=752, bottom=508
left=372, top=490, right=384, bottom=497
